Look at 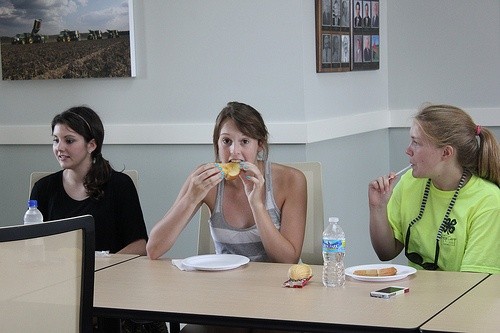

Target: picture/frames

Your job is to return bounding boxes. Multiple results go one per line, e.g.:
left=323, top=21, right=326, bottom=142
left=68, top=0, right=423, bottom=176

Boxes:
left=315, top=0, right=380, bottom=74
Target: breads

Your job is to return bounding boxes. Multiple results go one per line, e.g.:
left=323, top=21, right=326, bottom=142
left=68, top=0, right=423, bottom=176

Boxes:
left=288, top=264, right=312, bottom=280
left=354, top=267, right=396, bottom=277
left=218, top=162, right=242, bottom=180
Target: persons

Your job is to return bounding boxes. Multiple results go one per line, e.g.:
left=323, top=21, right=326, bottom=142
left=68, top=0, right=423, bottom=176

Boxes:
left=145, top=101, right=309, bottom=333
left=322, top=1, right=379, bottom=64
left=366, top=104, right=500, bottom=275
left=25, top=105, right=149, bottom=257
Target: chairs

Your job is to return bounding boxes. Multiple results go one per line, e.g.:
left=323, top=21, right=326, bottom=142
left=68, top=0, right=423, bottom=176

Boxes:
left=0, top=215, right=94, bottom=333
left=198, top=162, right=324, bottom=265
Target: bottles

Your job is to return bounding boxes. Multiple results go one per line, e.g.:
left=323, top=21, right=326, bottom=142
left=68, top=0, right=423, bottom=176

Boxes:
left=24, top=200, right=43, bottom=226
left=322, top=217, right=344, bottom=288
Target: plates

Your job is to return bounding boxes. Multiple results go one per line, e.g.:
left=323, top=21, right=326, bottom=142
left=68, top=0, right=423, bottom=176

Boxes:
left=182, top=253, right=250, bottom=271
left=344, top=263, right=417, bottom=281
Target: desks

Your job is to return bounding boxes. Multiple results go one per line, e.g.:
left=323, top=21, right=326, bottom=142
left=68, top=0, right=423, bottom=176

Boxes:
left=94, top=250, right=500, bottom=333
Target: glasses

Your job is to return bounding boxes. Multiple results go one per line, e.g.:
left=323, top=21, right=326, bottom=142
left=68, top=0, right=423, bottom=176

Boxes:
left=404, top=225, right=439, bottom=270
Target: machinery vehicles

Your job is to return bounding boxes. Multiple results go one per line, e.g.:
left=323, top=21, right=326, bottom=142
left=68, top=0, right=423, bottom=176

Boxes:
left=86, top=29, right=102, bottom=40
left=56, top=30, right=81, bottom=43
left=107, top=29, right=119, bottom=39
left=12, top=19, right=46, bottom=46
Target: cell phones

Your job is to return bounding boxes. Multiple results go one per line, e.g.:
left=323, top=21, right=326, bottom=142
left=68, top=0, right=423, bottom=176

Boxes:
left=370, top=286, right=409, bottom=299
